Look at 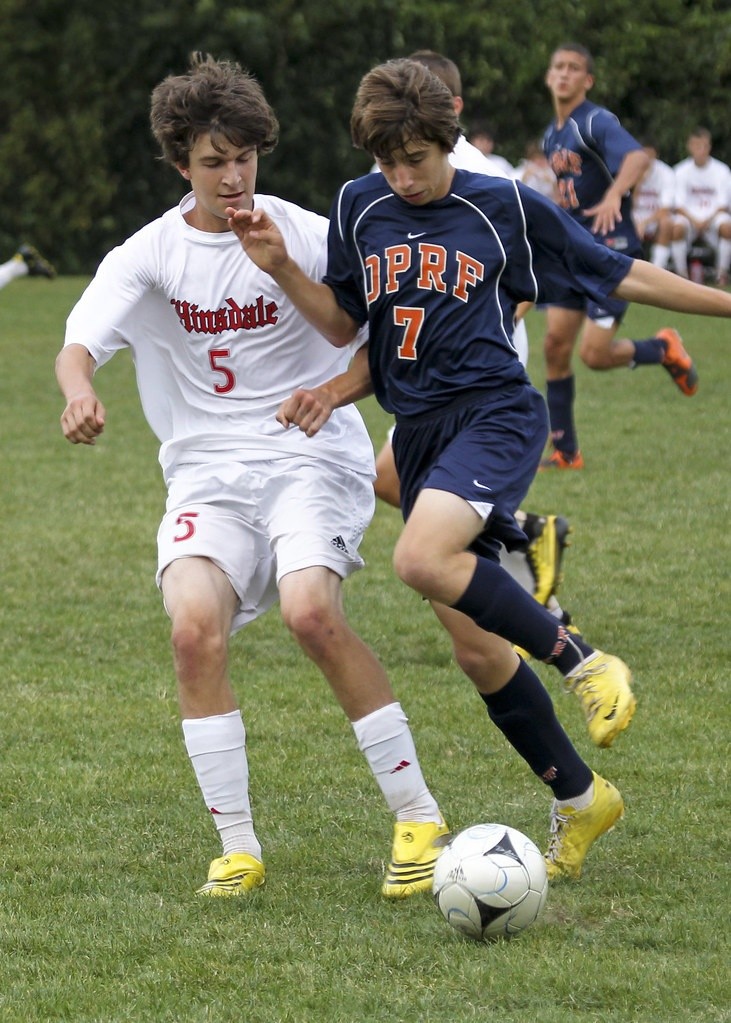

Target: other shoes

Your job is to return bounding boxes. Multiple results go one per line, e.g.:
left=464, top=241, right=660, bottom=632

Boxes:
left=512, top=625, right=580, bottom=661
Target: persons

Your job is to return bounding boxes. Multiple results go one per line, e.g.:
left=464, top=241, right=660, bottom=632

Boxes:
left=534, top=41, right=706, bottom=474
left=629, top=139, right=677, bottom=279
left=0, top=244, right=56, bottom=292
left=659, top=128, right=730, bottom=284
left=468, top=120, right=515, bottom=173
left=223, top=55, right=731, bottom=888
left=360, top=50, right=578, bottom=662
left=512, top=138, right=561, bottom=209
left=56, top=58, right=452, bottom=908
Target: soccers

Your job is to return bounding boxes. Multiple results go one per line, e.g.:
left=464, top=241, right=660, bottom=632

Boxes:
left=433, top=823, right=547, bottom=942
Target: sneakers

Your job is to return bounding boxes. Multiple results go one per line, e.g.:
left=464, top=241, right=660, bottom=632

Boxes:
left=382, top=816, right=453, bottom=897
left=540, top=770, right=623, bottom=882
left=658, top=326, right=701, bottom=395
left=536, top=451, right=587, bottom=471
left=566, top=649, right=640, bottom=748
left=193, top=853, right=266, bottom=899
left=526, top=516, right=569, bottom=609
